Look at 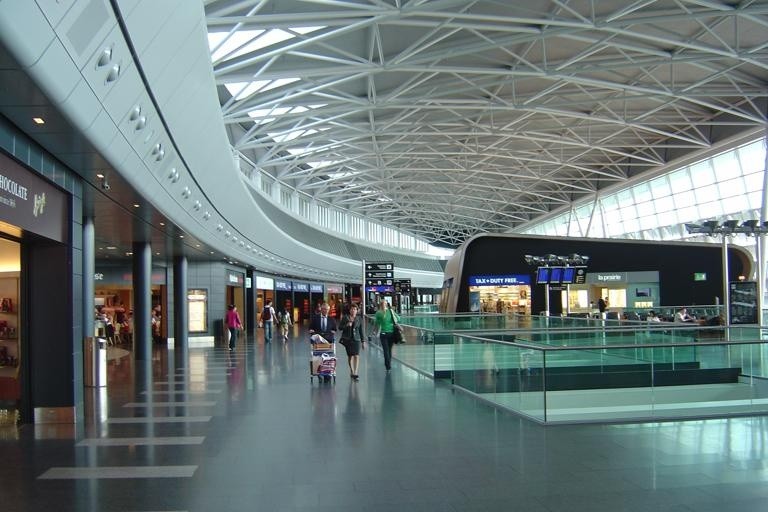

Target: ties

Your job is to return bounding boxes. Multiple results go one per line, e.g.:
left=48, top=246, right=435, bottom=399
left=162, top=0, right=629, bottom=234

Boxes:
left=322, top=316, right=324, bottom=333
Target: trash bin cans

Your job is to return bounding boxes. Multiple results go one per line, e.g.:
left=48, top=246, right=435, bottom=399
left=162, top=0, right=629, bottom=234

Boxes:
left=213, top=318, right=229, bottom=349
left=84, top=320, right=107, bottom=388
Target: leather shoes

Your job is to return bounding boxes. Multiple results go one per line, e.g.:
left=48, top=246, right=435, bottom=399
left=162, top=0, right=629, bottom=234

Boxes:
left=353, top=375, right=357, bottom=379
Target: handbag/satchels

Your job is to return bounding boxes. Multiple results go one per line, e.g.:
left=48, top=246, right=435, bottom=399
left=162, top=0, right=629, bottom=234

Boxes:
left=393, top=324, right=406, bottom=344
left=338, top=337, right=348, bottom=346
left=317, top=353, right=335, bottom=373
left=280, top=315, right=287, bottom=324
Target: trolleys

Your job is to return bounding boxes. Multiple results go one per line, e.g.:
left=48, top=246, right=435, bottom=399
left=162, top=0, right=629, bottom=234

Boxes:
left=310, top=330, right=336, bottom=384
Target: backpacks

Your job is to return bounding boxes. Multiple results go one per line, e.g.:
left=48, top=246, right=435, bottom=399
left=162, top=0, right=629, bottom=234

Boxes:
left=263, top=307, right=270, bottom=320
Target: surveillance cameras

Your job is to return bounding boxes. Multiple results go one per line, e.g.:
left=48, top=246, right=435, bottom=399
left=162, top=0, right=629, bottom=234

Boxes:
left=101, top=183, right=109, bottom=190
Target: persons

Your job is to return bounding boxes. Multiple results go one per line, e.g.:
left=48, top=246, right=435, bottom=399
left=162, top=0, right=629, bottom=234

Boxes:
left=308, top=302, right=337, bottom=384
left=104, top=314, right=113, bottom=347
left=673, top=306, right=699, bottom=343
left=276, top=307, right=294, bottom=342
left=518, top=298, right=528, bottom=315
left=225, top=304, right=244, bottom=351
left=314, top=298, right=362, bottom=329
left=259, top=300, right=280, bottom=344
left=598, top=298, right=607, bottom=312
left=372, top=300, right=402, bottom=372
left=646, top=311, right=661, bottom=322
left=338, top=302, right=367, bottom=379
